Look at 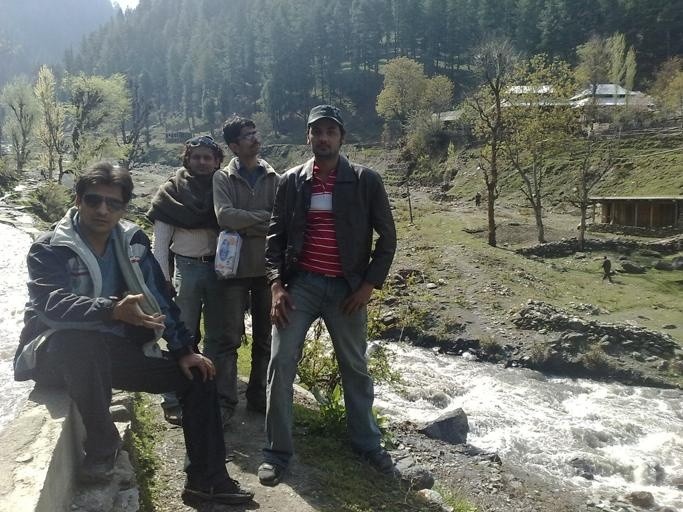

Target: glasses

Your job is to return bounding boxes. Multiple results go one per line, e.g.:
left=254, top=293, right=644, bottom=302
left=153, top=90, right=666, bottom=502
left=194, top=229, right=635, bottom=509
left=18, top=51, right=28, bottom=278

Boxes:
left=84, top=194, right=123, bottom=211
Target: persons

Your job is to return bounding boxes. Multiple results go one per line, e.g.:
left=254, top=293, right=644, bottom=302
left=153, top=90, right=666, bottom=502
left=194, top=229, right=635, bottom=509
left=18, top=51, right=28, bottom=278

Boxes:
left=211, top=115, right=282, bottom=433
left=257, top=105, right=396, bottom=486
left=144, top=136, right=226, bottom=427
left=601, top=256, right=615, bottom=283
left=12, top=161, right=256, bottom=507
left=475, top=191, right=482, bottom=206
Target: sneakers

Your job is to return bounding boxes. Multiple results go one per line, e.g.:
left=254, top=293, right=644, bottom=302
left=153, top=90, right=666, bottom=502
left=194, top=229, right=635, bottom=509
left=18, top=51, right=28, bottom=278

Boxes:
left=184, top=477, right=254, bottom=504
left=258, top=462, right=284, bottom=485
left=81, top=439, right=118, bottom=482
left=353, top=445, right=393, bottom=473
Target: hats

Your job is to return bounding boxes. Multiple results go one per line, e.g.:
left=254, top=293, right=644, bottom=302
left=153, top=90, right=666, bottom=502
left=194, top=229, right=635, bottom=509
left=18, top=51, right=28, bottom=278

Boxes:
left=308, top=105, right=343, bottom=126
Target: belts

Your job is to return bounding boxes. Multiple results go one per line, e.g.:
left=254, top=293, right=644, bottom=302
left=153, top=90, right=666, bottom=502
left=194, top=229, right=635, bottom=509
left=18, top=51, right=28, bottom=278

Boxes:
left=177, top=254, right=215, bottom=262
